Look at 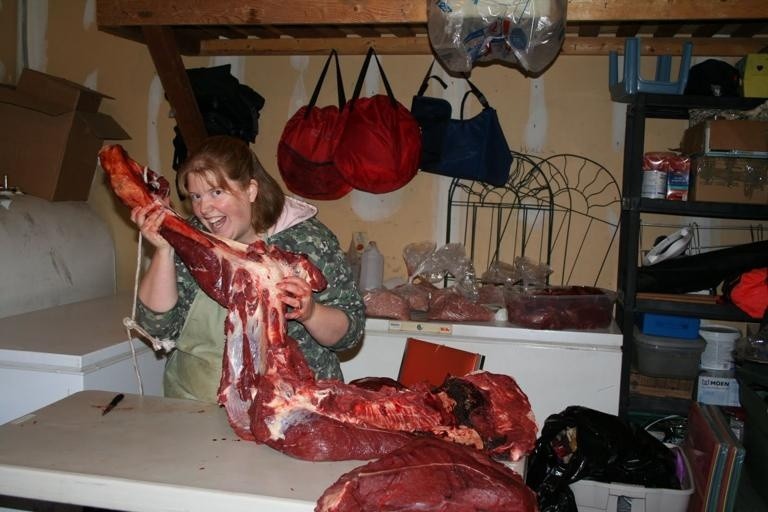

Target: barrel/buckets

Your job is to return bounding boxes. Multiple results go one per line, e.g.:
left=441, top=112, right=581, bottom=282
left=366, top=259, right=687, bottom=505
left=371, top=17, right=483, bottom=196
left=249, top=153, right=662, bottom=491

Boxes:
left=699, top=324, right=742, bottom=372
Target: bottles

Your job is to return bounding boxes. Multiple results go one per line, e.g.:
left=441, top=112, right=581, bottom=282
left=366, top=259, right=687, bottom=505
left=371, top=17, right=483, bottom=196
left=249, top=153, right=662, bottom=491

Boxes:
left=346, top=231, right=366, bottom=291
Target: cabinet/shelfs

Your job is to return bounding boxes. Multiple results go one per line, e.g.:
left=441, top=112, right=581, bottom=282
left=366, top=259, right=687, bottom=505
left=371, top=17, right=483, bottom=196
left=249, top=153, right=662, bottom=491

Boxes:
left=617, top=98, right=768, bottom=420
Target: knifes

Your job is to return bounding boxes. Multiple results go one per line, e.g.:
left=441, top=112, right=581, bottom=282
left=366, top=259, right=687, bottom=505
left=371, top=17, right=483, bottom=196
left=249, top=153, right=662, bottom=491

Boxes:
left=101, top=392, right=125, bottom=416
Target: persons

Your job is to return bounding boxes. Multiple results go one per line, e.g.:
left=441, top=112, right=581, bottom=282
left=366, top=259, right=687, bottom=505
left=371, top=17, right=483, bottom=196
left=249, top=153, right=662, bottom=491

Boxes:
left=129, top=135, right=366, bottom=406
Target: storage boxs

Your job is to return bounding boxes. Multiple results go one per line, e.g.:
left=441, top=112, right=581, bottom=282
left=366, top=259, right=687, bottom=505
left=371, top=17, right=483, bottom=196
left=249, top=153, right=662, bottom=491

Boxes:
left=679, top=118, right=768, bottom=159
left=0, top=66, right=131, bottom=201
left=568, top=443, right=695, bottom=512
left=627, top=314, right=741, bottom=419
left=689, top=155, right=767, bottom=205
left=734, top=53, right=768, bottom=99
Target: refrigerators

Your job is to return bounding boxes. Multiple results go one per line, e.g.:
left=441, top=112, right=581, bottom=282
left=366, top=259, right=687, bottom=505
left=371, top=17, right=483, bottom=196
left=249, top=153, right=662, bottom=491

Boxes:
left=0, top=289, right=180, bottom=425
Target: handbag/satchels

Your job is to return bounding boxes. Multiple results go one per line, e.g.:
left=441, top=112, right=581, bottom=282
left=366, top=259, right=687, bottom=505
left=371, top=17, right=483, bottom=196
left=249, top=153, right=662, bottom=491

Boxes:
left=274, top=46, right=422, bottom=202
left=412, top=53, right=514, bottom=190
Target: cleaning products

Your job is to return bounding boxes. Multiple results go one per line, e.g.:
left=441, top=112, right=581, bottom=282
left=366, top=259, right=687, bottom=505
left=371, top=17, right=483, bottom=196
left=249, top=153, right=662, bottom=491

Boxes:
left=347, top=233, right=366, bottom=264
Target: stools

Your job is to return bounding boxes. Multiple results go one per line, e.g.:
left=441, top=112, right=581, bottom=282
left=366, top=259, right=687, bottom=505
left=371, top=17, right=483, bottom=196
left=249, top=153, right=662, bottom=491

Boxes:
left=609, top=38, right=693, bottom=103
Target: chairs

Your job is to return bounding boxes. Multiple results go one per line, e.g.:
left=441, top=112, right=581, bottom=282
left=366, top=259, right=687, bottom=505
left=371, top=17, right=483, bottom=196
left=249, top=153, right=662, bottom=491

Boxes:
left=396, top=338, right=486, bottom=394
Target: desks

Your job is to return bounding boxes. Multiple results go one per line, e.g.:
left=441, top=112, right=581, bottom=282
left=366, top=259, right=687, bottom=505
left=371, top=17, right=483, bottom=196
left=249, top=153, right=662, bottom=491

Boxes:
left=733, top=348, right=768, bottom=506
left=0, top=389, right=528, bottom=512
